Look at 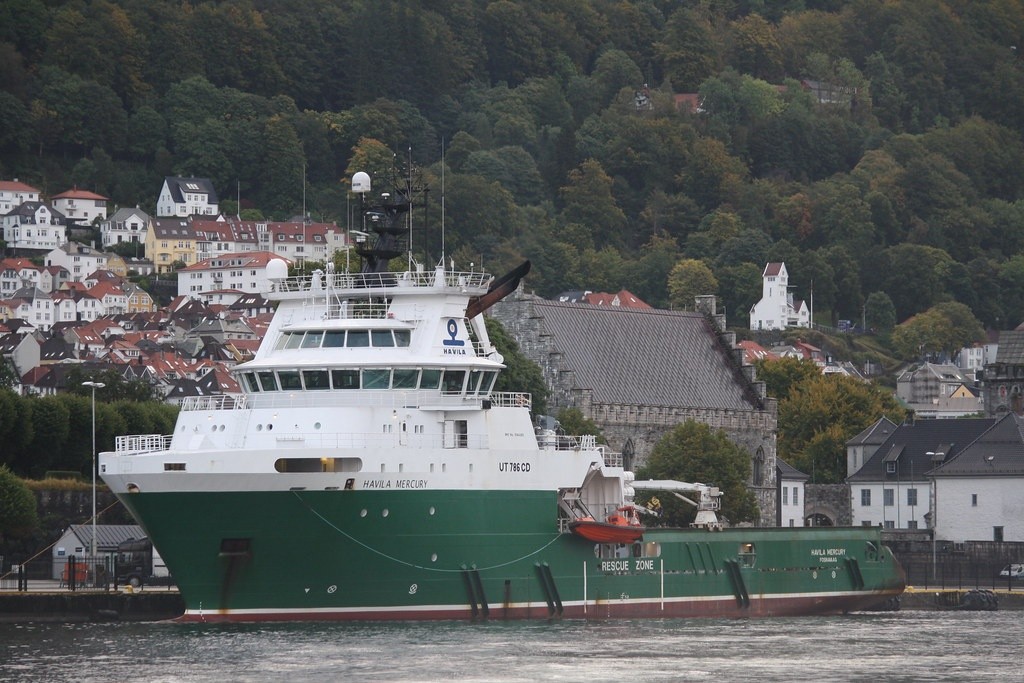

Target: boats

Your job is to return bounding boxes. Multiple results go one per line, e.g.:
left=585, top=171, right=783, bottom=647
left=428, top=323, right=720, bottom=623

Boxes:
left=569, top=509, right=645, bottom=546
left=97, top=138, right=908, bottom=626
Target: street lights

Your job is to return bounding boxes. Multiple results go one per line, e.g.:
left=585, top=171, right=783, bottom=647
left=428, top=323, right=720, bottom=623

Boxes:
left=83, top=381, right=107, bottom=588
left=927, top=452, right=944, bottom=579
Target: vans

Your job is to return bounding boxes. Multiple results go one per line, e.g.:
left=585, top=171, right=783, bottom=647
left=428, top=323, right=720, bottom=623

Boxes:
left=1000, top=564, right=1024, bottom=581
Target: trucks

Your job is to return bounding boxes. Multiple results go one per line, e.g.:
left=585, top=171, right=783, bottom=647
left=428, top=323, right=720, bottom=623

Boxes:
left=115, top=535, right=174, bottom=587
